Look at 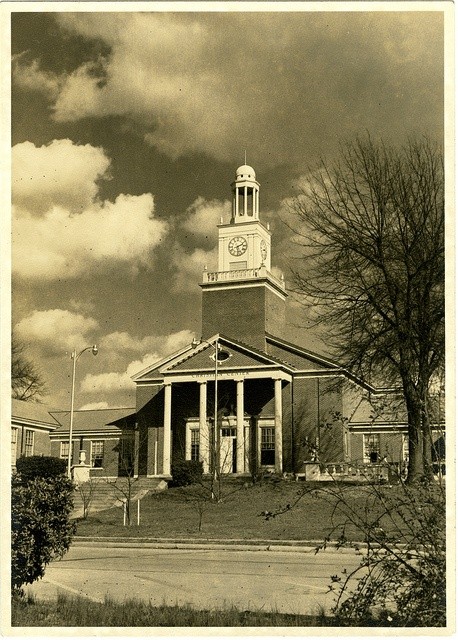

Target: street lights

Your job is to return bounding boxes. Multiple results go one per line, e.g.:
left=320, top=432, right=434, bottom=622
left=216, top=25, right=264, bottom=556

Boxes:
left=191, top=336, right=219, bottom=480
left=67, top=343, right=98, bottom=480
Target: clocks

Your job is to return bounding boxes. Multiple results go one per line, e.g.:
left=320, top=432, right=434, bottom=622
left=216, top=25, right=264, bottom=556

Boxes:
left=227, top=235, right=248, bottom=258
left=259, top=238, right=268, bottom=261
